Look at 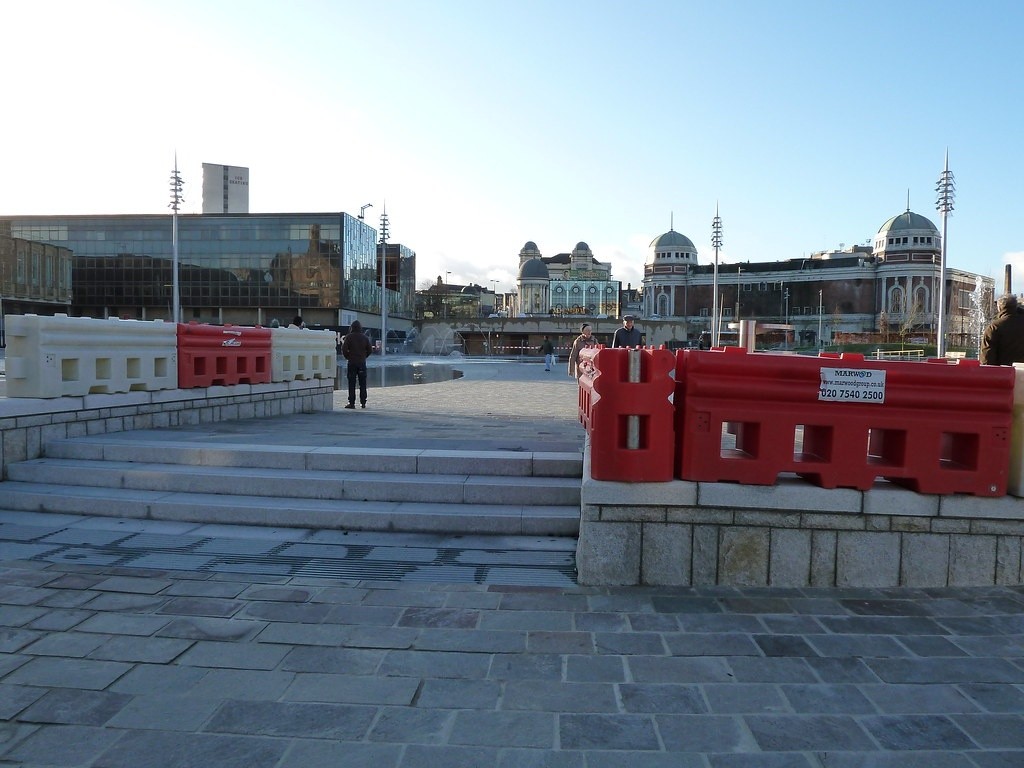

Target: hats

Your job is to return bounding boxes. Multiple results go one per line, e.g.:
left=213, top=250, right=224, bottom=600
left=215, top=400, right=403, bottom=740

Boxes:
left=623, top=315, right=635, bottom=320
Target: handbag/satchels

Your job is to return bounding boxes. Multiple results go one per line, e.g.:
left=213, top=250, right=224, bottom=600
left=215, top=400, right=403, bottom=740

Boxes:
left=551, top=355, right=555, bottom=365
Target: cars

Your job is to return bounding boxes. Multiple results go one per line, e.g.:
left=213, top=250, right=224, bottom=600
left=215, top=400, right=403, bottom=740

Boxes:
left=515, top=312, right=533, bottom=318
left=650, top=314, right=661, bottom=320
left=912, top=338, right=928, bottom=344
left=424, top=312, right=434, bottom=319
left=591, top=314, right=608, bottom=319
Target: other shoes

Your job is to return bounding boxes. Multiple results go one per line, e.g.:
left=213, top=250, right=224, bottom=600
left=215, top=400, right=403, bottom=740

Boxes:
left=360, top=404, right=366, bottom=408
left=344, top=403, right=355, bottom=408
left=545, top=369, right=550, bottom=371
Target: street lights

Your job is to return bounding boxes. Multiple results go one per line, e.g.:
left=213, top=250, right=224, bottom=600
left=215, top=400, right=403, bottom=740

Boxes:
left=783, top=288, right=788, bottom=350
left=444, top=270, right=451, bottom=318
left=490, top=280, right=499, bottom=309
left=167, top=149, right=186, bottom=323
left=738, top=267, right=746, bottom=302
left=711, top=200, right=724, bottom=347
left=873, top=253, right=879, bottom=331
left=379, top=201, right=390, bottom=355
left=685, top=262, right=690, bottom=316
left=933, top=147, right=956, bottom=358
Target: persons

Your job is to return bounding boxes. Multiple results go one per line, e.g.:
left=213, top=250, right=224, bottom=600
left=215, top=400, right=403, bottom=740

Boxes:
left=612, top=315, right=643, bottom=349
left=568, top=323, right=599, bottom=384
left=537, top=335, right=553, bottom=371
left=980, top=294, right=1024, bottom=366
left=288, top=316, right=303, bottom=330
left=699, top=336, right=704, bottom=350
left=341, top=320, right=372, bottom=409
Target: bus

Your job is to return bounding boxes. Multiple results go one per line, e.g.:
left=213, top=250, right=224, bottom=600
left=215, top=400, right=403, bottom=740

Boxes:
left=699, top=332, right=738, bottom=345
